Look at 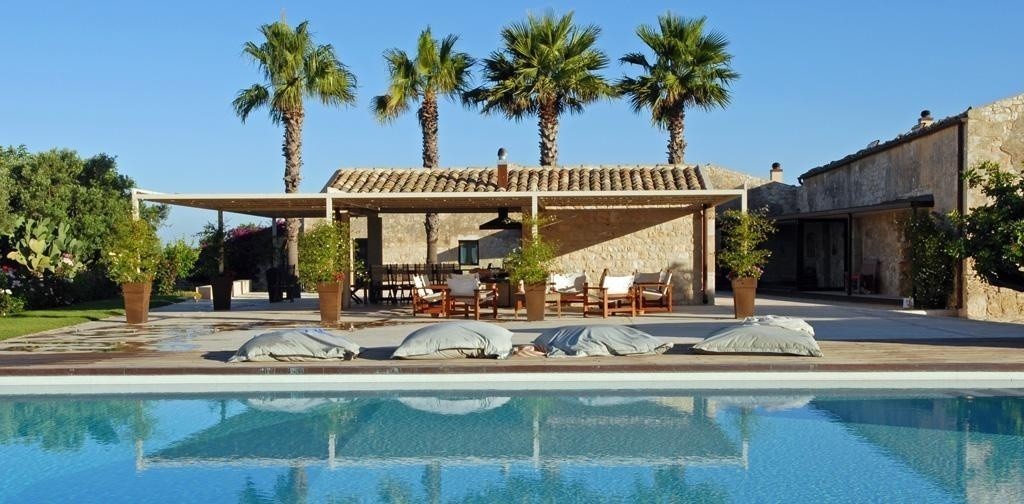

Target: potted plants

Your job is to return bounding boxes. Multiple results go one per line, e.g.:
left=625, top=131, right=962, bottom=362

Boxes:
left=298, top=217, right=364, bottom=321
left=106, top=222, right=163, bottom=323
left=503, top=211, right=559, bottom=321
left=719, top=205, right=781, bottom=320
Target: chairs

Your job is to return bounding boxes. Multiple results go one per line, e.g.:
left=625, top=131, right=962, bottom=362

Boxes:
left=352, top=263, right=499, bottom=319
left=514, top=264, right=674, bottom=318
